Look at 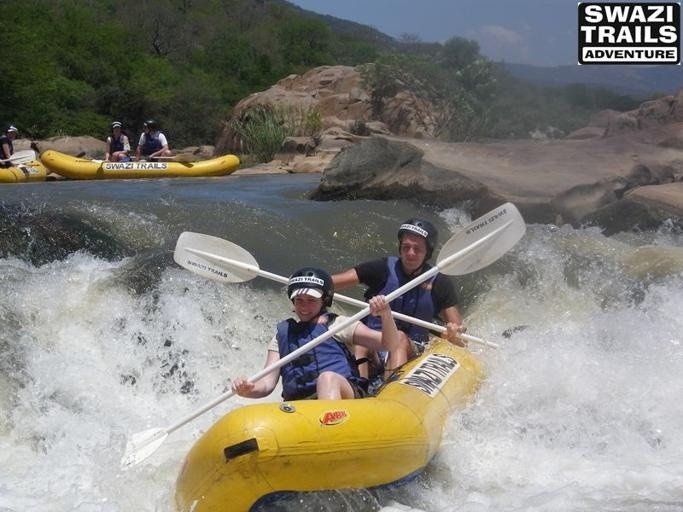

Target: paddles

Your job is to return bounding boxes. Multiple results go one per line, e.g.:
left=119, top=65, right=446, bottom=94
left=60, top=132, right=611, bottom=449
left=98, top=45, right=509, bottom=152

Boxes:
left=0, top=150, right=35, bottom=164
left=130, top=153, right=196, bottom=162
left=120, top=201, right=526, bottom=472
left=173, top=230, right=500, bottom=349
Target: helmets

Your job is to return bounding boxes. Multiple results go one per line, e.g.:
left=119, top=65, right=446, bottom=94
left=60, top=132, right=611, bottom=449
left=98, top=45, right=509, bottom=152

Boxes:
left=6, top=124, right=19, bottom=134
left=397, top=216, right=439, bottom=263
left=111, top=120, right=124, bottom=134
left=144, top=119, right=161, bottom=131
left=287, top=267, right=335, bottom=310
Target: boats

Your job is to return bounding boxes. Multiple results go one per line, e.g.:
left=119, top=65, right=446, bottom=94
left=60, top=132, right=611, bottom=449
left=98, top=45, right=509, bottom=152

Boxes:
left=38, top=148, right=243, bottom=179
left=0, top=158, right=49, bottom=183
left=170, top=329, right=489, bottom=512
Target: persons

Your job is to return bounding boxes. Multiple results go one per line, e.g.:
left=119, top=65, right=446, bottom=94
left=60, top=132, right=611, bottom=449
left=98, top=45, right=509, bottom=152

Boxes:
left=231, top=267, right=400, bottom=399
left=331, top=218, right=465, bottom=382
left=105, top=121, right=130, bottom=161
left=0, top=125, right=18, bottom=168
left=136, top=119, right=172, bottom=162
